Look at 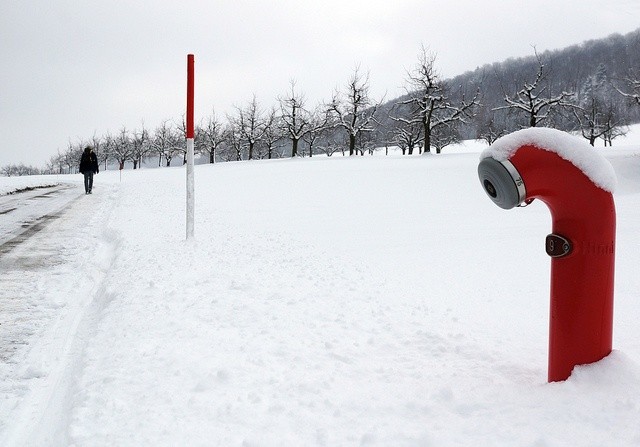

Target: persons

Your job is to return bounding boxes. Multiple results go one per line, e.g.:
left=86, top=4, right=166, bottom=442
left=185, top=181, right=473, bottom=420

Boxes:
left=79, top=147, right=99, bottom=195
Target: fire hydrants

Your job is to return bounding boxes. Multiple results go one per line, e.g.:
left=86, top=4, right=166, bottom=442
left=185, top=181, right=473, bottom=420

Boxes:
left=478, top=134, right=618, bottom=382
